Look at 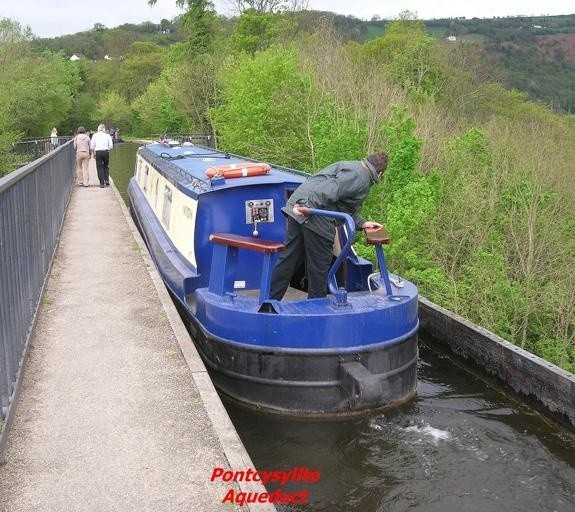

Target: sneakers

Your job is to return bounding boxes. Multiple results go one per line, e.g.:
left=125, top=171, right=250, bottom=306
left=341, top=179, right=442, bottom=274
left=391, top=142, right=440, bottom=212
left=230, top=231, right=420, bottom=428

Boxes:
left=78, top=182, right=89, bottom=187
left=100, top=181, right=110, bottom=188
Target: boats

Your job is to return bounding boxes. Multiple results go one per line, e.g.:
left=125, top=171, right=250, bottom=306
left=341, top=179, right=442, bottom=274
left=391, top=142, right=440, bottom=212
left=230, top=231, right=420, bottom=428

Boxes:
left=126, top=138, right=419, bottom=423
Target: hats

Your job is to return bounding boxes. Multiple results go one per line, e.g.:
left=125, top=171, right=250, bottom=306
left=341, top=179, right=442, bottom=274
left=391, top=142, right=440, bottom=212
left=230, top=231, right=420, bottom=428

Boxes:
left=367, top=153, right=389, bottom=178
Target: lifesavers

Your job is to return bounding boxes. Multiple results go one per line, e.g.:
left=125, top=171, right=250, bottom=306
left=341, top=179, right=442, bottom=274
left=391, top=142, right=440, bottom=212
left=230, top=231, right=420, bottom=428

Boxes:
left=206, top=163, right=272, bottom=178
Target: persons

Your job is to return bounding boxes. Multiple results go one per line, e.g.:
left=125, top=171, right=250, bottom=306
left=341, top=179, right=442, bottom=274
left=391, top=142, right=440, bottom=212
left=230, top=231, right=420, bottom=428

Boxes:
left=264, top=150, right=389, bottom=302
left=107, top=127, right=121, bottom=139
left=89, top=123, right=114, bottom=190
left=74, top=126, right=91, bottom=188
left=85, top=129, right=95, bottom=160
left=50, top=127, right=59, bottom=150
